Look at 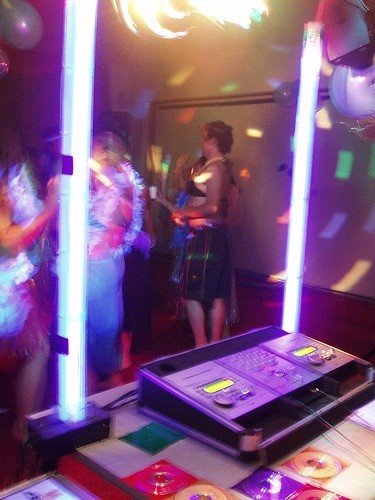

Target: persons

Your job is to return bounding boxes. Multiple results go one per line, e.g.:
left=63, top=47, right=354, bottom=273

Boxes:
left=0, top=120, right=241, bottom=455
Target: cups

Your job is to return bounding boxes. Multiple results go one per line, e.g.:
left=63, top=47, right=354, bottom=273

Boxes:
left=149, top=186, right=157, bottom=199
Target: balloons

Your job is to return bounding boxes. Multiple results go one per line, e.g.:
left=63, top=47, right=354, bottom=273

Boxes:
left=274, top=79, right=300, bottom=109
left=0, top=0, right=48, bottom=83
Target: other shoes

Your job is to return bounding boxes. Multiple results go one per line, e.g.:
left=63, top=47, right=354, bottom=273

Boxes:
left=130, top=337, right=153, bottom=354
